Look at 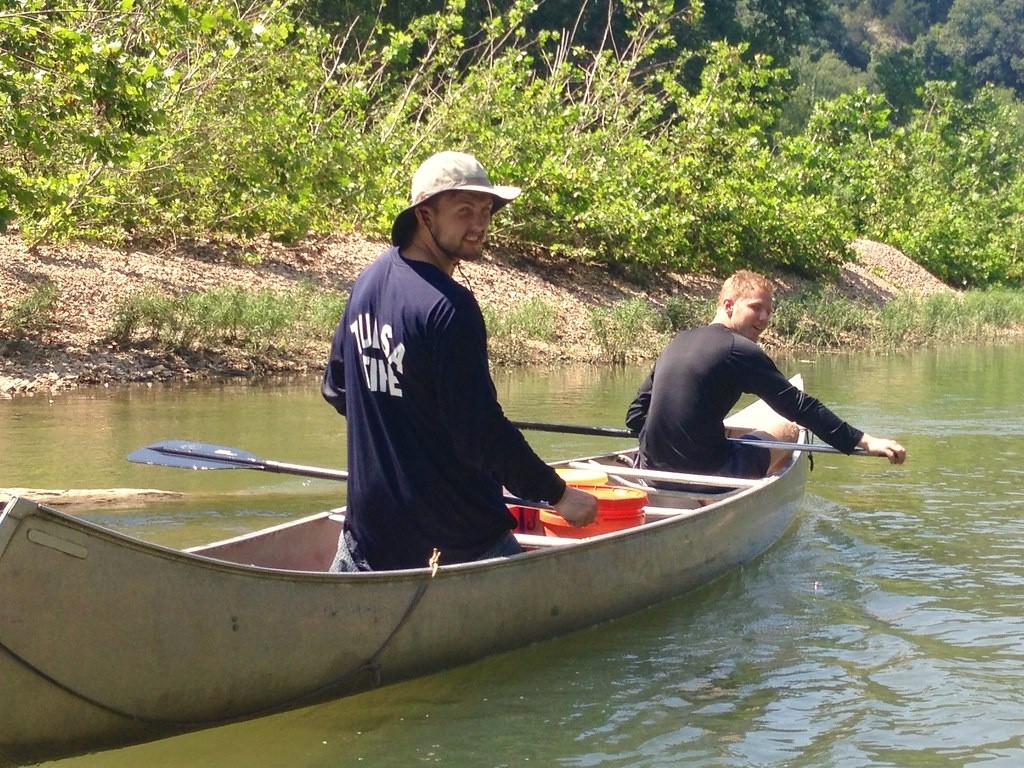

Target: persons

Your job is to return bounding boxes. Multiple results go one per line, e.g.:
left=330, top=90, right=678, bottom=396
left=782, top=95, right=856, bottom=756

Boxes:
left=319, top=152, right=600, bottom=577
left=627, top=269, right=906, bottom=480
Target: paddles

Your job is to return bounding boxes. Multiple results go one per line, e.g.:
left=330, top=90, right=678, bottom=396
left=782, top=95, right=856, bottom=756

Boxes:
left=512, top=420, right=888, bottom=457
left=127, top=438, right=561, bottom=511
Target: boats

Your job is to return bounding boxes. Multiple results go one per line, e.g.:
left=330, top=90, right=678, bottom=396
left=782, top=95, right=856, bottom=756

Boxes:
left=1, top=374, right=810, bottom=768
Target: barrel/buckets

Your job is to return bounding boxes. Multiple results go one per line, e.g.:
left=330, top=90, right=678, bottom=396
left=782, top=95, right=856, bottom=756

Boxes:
left=504, top=469, right=609, bottom=552
left=539, top=484, right=649, bottom=539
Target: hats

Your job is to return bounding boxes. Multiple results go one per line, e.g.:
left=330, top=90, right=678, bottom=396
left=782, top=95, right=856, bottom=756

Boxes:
left=392, top=151, right=522, bottom=247
